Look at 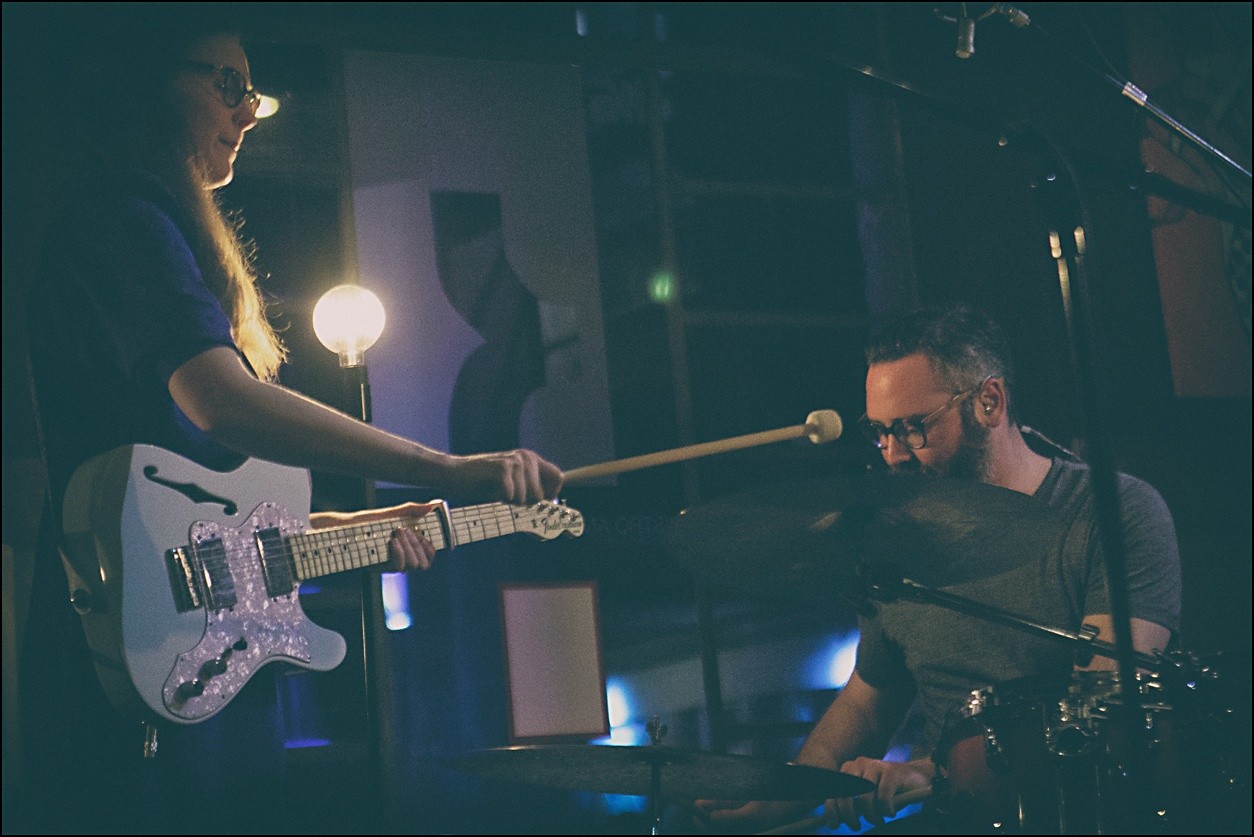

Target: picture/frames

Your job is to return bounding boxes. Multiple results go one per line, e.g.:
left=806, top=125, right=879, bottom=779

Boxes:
left=498, top=580, right=611, bottom=746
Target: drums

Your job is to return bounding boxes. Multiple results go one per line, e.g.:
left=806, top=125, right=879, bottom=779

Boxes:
left=929, top=668, right=1254, bottom=836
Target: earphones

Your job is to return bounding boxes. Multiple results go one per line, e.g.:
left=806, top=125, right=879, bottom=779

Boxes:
left=985, top=405, right=995, bottom=414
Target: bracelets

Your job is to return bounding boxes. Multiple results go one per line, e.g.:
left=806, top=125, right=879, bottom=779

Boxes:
left=980, top=726, right=1004, bottom=764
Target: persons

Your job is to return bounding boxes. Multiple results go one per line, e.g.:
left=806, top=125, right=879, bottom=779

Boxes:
left=695, top=306, right=1184, bottom=837
left=18, top=0, right=567, bottom=837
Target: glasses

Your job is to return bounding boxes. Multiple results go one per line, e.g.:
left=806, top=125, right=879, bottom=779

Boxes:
left=856, top=384, right=982, bottom=449
left=175, top=59, right=260, bottom=115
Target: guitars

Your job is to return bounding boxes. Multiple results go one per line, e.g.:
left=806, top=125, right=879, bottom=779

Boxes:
left=56, top=442, right=585, bottom=728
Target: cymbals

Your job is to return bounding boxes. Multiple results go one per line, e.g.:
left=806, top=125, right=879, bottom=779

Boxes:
left=646, top=469, right=1062, bottom=594
left=442, top=741, right=878, bottom=803
